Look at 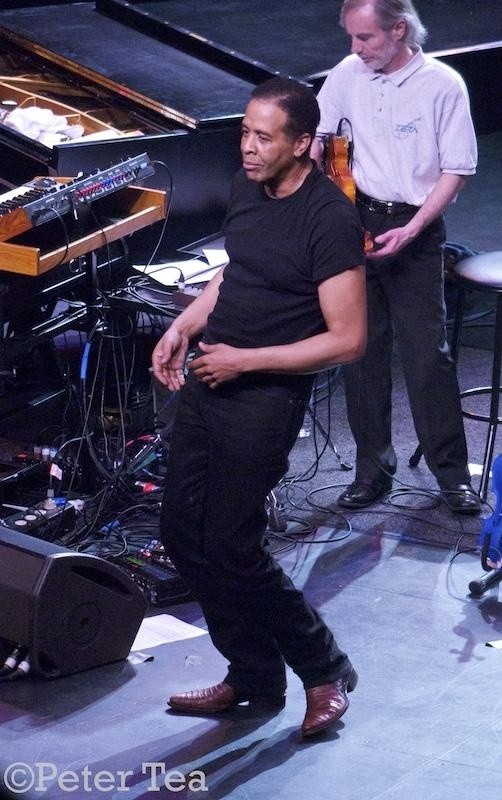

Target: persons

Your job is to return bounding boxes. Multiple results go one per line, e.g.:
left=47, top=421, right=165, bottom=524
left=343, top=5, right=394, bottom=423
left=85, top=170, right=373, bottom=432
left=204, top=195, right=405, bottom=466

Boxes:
left=151, top=78, right=369, bottom=737
left=309, top=0, right=480, bottom=512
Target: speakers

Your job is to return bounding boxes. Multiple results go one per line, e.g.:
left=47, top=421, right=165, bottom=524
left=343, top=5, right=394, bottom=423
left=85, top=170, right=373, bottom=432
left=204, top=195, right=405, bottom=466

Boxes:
left=0, top=528, right=153, bottom=682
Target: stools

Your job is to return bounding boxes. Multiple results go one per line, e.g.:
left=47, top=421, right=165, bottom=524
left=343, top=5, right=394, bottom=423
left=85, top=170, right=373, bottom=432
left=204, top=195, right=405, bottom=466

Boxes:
left=409, top=250, right=502, bottom=504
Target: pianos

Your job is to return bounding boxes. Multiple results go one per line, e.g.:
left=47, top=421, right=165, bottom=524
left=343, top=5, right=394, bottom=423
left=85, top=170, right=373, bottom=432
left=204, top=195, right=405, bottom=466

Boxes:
left=1, top=152, right=156, bottom=242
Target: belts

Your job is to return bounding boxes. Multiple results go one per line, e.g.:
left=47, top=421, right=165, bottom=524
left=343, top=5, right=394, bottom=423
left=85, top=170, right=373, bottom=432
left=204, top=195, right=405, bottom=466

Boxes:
left=357, top=194, right=407, bottom=214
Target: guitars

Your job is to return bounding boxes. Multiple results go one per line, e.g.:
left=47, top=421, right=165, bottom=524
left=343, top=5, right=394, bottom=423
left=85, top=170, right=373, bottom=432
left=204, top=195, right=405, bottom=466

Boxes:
left=322, top=133, right=373, bottom=252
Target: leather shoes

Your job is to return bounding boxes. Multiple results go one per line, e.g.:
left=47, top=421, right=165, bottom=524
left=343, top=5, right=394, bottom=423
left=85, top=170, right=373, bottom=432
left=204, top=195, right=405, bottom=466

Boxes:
left=338, top=477, right=392, bottom=507
left=302, top=666, right=358, bottom=737
left=441, top=483, right=481, bottom=512
left=167, top=679, right=286, bottom=713
